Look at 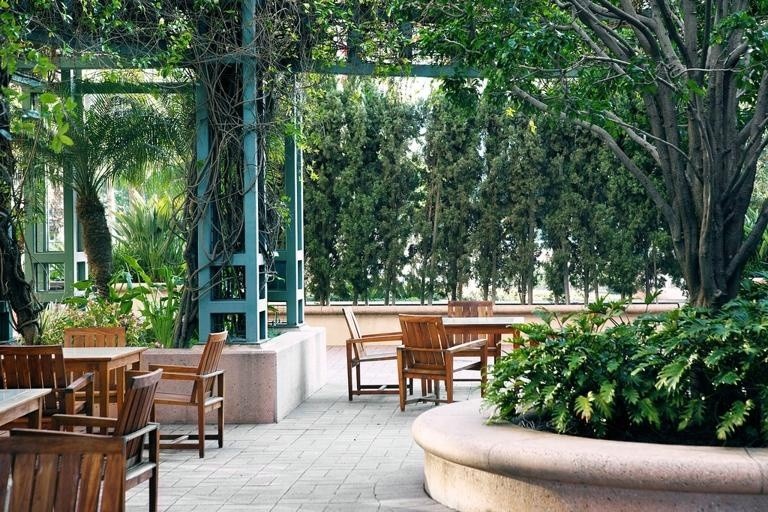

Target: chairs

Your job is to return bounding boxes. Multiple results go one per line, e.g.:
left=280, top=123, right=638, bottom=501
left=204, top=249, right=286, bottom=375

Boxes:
left=341, top=291, right=493, bottom=411
left=0, top=435, right=124, bottom=512
left=10, top=368, right=163, bottom=512
left=60, top=326, right=127, bottom=419
left=147, top=330, right=228, bottom=459
left=1, top=344, right=95, bottom=433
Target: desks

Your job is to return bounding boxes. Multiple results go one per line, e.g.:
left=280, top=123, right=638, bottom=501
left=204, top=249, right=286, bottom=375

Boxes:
left=3, top=346, right=147, bottom=433
left=1, top=388, right=52, bottom=512
left=443, top=314, right=526, bottom=359
